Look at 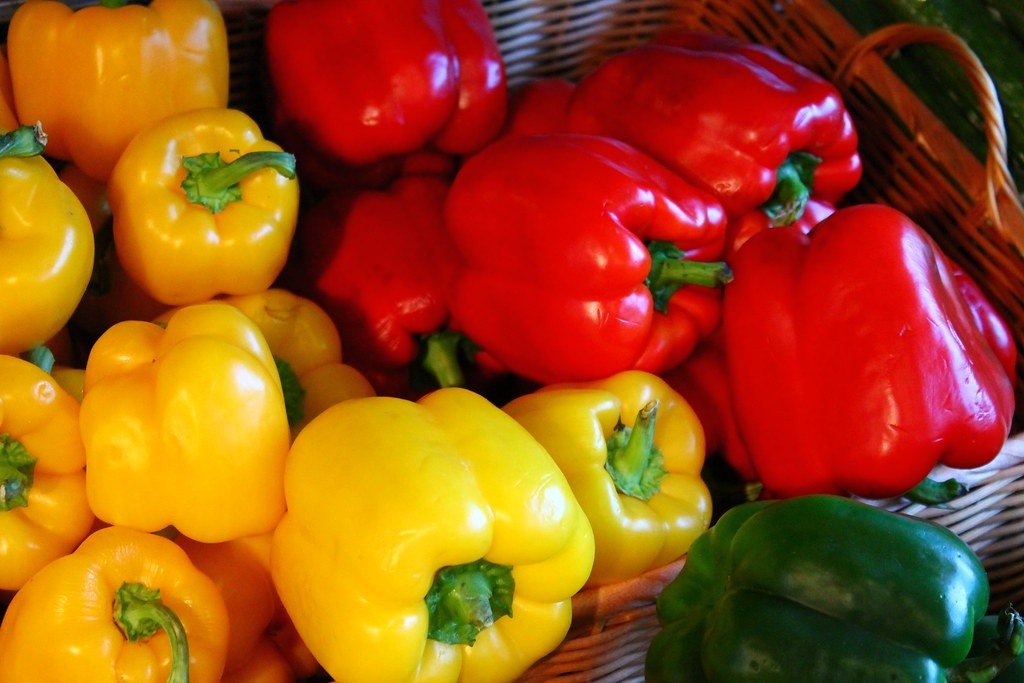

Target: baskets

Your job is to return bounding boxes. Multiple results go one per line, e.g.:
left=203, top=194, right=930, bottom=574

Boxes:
left=51, top=1, right=1024, bottom=683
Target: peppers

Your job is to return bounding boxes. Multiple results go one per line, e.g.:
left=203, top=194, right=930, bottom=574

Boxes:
left=0, top=0, right=1018, bottom=683
